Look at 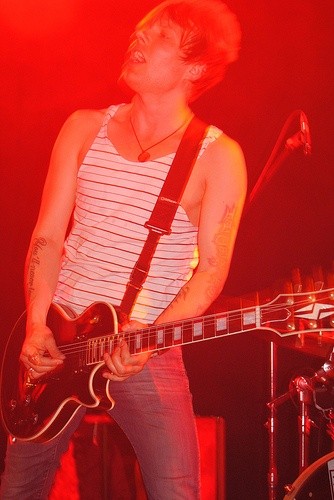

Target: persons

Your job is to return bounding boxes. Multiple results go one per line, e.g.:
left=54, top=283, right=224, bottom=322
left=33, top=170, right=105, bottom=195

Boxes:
left=0, top=1, right=248, bottom=500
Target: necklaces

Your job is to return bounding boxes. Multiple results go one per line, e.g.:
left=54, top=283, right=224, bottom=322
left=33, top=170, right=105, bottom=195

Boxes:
left=130, top=106, right=193, bottom=161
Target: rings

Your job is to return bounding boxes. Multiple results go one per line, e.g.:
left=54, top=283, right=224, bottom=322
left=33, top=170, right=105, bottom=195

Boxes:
left=29, top=354, right=40, bottom=365
left=28, top=368, right=34, bottom=374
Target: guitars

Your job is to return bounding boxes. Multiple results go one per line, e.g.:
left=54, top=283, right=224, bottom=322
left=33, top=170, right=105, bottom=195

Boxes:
left=0, top=279, right=334, bottom=444
